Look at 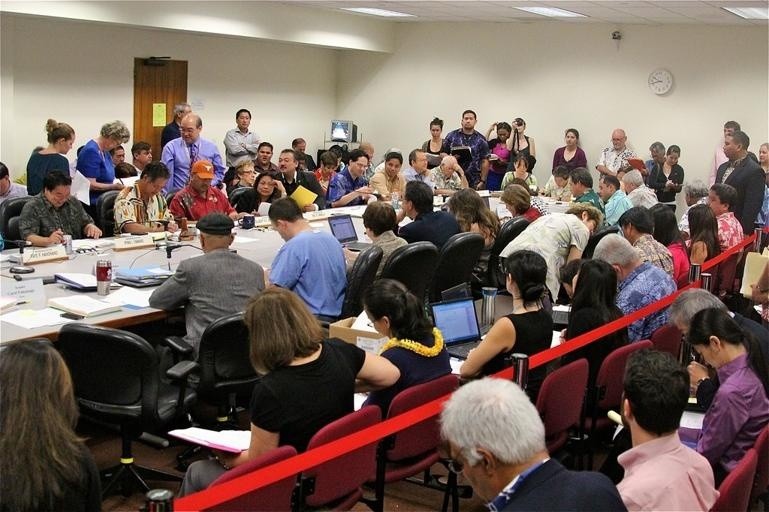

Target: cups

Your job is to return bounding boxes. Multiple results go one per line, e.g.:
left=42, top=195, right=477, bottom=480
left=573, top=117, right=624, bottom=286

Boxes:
left=238, top=214, right=255, bottom=229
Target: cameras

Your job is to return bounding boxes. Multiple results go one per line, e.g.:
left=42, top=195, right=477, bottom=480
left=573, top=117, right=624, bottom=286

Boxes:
left=515, top=120, right=524, bottom=126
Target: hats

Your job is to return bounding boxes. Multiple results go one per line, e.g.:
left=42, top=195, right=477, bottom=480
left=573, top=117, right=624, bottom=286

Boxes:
left=196, top=214, right=233, bottom=235
left=191, top=160, right=214, bottom=179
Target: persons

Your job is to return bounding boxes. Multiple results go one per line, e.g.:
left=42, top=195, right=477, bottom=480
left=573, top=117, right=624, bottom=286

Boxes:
left=0, top=102, right=769, bottom=512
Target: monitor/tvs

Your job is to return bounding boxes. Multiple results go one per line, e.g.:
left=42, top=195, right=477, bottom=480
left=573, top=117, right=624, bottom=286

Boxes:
left=330, top=119, right=358, bottom=143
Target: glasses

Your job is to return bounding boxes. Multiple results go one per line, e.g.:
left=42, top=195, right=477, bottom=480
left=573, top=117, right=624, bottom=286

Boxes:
left=448, top=446, right=464, bottom=474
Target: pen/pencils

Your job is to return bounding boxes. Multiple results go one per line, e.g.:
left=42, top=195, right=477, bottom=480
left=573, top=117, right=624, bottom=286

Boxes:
left=118, top=177, right=124, bottom=185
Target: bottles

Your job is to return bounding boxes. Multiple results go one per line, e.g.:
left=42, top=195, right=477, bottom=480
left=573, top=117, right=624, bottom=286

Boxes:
left=95, top=261, right=113, bottom=296
left=391, top=188, right=399, bottom=210
left=62, top=234, right=74, bottom=255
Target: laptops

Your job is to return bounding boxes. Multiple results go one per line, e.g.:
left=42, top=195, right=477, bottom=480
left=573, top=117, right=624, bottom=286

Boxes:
left=327, top=215, right=372, bottom=252
left=541, top=290, right=570, bottom=326
left=429, top=296, right=483, bottom=360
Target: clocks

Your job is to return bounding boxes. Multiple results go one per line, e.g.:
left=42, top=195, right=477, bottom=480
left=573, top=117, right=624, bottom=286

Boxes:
left=648, top=68, right=674, bottom=95
